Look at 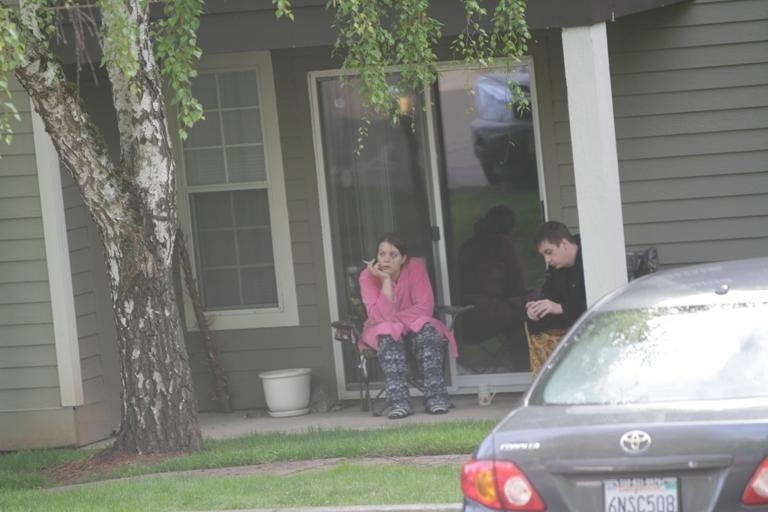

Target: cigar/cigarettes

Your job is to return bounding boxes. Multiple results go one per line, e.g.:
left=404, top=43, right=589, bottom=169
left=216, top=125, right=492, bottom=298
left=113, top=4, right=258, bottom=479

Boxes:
left=361, top=259, right=369, bottom=264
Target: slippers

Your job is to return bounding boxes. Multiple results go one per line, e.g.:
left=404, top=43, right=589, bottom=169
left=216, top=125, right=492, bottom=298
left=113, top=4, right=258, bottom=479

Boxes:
left=425, top=403, right=448, bottom=414
left=388, top=408, right=415, bottom=418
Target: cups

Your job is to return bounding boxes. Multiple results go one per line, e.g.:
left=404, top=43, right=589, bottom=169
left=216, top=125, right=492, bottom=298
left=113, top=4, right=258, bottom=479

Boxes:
left=478, top=384, right=496, bottom=406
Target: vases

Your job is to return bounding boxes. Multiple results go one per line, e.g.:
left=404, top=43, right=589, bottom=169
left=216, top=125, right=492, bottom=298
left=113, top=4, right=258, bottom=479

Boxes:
left=260, top=365, right=313, bottom=418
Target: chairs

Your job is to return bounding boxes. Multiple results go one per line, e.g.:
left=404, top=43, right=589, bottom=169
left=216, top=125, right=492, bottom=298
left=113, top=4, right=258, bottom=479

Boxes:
left=521, top=249, right=657, bottom=378
left=330, top=265, right=464, bottom=418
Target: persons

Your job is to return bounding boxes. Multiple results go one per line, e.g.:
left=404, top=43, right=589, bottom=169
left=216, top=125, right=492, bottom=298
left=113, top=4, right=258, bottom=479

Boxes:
left=358, top=233, right=459, bottom=419
left=521, top=220, right=587, bottom=380
left=454, top=204, right=542, bottom=373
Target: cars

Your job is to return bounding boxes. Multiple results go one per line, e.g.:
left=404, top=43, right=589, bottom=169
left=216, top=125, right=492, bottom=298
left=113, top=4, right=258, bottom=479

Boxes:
left=460, top=257, right=768, bottom=512
